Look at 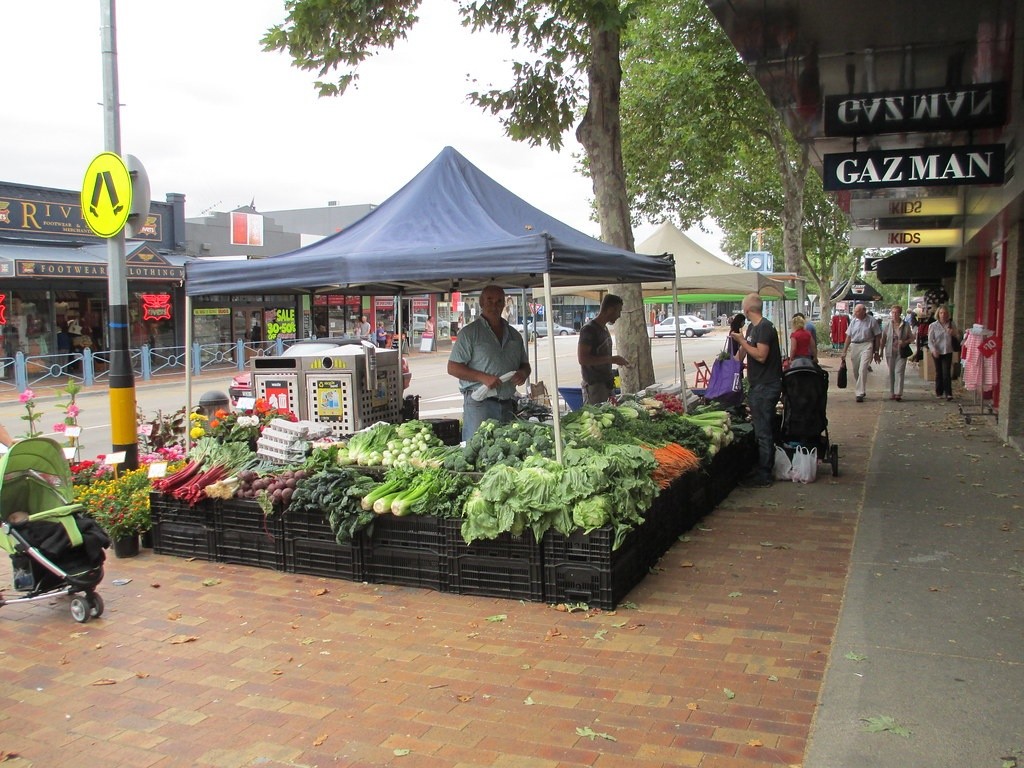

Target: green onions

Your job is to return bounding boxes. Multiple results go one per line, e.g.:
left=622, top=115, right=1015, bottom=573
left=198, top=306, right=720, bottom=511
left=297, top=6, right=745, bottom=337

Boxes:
left=411, top=443, right=462, bottom=470
left=204, top=458, right=279, bottom=500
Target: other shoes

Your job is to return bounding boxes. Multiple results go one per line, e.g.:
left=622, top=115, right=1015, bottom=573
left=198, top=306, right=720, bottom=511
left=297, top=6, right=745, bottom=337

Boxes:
left=748, top=478, right=771, bottom=488
left=890, top=394, right=901, bottom=401
left=856, top=392, right=866, bottom=401
left=937, top=394, right=943, bottom=399
left=947, top=395, right=953, bottom=401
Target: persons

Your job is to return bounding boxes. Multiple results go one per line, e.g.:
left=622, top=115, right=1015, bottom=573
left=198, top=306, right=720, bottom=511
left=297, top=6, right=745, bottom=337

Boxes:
left=58, top=329, right=70, bottom=372
left=577, top=293, right=629, bottom=406
left=465, top=298, right=479, bottom=322
left=728, top=294, right=784, bottom=487
left=446, top=285, right=531, bottom=442
left=377, top=321, right=387, bottom=348
left=727, top=314, right=748, bottom=378
left=880, top=306, right=915, bottom=400
left=882, top=316, right=890, bottom=328
left=360, top=316, right=370, bottom=334
left=842, top=304, right=882, bottom=402
left=506, top=297, right=516, bottom=324
left=553, top=312, right=560, bottom=324
left=790, top=313, right=818, bottom=367
left=929, top=305, right=959, bottom=400
left=425, top=316, right=433, bottom=332
left=907, top=308, right=919, bottom=334
left=913, top=303, right=922, bottom=314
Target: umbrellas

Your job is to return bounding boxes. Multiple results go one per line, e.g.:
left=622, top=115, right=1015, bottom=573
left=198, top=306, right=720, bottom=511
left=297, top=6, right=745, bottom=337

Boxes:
left=832, top=275, right=883, bottom=310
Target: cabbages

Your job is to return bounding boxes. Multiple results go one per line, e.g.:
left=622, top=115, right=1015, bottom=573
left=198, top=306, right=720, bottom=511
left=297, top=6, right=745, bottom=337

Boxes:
left=462, top=444, right=661, bottom=547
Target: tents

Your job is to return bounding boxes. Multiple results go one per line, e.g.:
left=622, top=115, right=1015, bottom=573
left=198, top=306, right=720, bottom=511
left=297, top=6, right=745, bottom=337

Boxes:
left=533, top=219, right=789, bottom=384
left=184, top=146, right=687, bottom=464
left=644, top=286, right=799, bottom=356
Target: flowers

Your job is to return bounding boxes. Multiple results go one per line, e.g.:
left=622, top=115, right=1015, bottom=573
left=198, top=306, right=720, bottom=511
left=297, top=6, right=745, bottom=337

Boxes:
left=14, top=379, right=296, bottom=541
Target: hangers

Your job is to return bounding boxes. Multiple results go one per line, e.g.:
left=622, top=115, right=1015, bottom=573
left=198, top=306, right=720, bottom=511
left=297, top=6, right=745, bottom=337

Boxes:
left=968, top=324, right=994, bottom=336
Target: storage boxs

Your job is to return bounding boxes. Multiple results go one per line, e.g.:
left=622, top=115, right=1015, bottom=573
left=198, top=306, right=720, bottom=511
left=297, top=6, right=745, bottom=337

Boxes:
left=149, top=414, right=783, bottom=611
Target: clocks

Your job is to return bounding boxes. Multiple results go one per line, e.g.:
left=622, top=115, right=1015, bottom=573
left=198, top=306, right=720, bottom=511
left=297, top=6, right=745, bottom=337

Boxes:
left=767, top=257, right=772, bottom=269
left=750, top=257, right=762, bottom=269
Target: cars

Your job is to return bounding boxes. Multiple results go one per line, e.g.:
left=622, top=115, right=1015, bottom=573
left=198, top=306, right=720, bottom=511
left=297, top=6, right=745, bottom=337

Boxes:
left=655, top=314, right=715, bottom=338
left=229, top=336, right=412, bottom=408
left=509, top=323, right=532, bottom=342
left=527, top=321, right=576, bottom=338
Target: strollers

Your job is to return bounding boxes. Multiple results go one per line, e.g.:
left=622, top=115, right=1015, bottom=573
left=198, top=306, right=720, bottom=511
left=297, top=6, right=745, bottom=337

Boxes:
left=0, top=436, right=113, bottom=624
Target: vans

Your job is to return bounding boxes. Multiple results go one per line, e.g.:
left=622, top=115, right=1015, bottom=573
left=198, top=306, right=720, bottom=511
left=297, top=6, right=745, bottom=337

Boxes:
left=392, top=313, right=450, bottom=337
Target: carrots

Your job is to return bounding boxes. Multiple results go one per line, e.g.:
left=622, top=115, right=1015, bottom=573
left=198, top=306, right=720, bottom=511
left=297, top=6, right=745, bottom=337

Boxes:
left=642, top=443, right=701, bottom=491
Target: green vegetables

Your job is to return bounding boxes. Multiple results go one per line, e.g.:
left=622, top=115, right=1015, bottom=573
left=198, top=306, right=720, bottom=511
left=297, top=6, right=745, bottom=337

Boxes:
left=295, top=421, right=443, bottom=469
left=717, top=350, right=731, bottom=362
left=562, top=376, right=749, bottom=464
left=151, top=438, right=256, bottom=503
left=283, top=460, right=473, bottom=545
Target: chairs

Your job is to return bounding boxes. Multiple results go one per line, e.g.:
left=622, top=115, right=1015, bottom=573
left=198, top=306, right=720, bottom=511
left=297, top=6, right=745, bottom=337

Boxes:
left=693, top=360, right=711, bottom=388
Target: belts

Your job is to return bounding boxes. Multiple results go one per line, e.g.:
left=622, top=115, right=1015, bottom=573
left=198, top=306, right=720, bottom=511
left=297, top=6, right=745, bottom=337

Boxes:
left=488, top=397, right=510, bottom=405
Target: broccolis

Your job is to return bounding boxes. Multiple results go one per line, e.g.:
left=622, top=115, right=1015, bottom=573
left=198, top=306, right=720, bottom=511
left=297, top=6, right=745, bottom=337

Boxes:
left=444, top=455, right=456, bottom=470
left=463, top=418, right=558, bottom=470
left=454, top=457, right=474, bottom=471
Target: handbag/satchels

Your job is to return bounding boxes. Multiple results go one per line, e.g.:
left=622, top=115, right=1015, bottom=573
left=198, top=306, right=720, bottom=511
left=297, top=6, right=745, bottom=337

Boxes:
left=772, top=445, right=793, bottom=480
left=704, top=335, right=743, bottom=403
left=68, top=319, right=82, bottom=336
left=837, top=357, right=847, bottom=389
left=949, top=320, right=962, bottom=352
left=793, top=445, right=817, bottom=485
left=899, top=322, right=913, bottom=358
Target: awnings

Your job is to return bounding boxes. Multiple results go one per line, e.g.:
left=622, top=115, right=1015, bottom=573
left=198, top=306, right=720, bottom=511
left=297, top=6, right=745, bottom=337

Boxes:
left=877, top=247, right=955, bottom=285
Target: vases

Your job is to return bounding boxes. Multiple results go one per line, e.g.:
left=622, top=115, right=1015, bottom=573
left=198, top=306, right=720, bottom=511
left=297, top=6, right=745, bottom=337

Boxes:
left=141, top=530, right=153, bottom=548
left=113, top=532, right=139, bottom=558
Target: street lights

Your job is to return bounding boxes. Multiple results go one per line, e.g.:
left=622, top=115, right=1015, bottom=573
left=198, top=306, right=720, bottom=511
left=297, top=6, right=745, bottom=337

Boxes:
left=807, top=291, right=818, bottom=322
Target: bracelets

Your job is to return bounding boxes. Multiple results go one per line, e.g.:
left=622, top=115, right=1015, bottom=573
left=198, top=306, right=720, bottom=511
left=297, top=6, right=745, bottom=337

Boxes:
left=874, top=351, right=879, bottom=353
